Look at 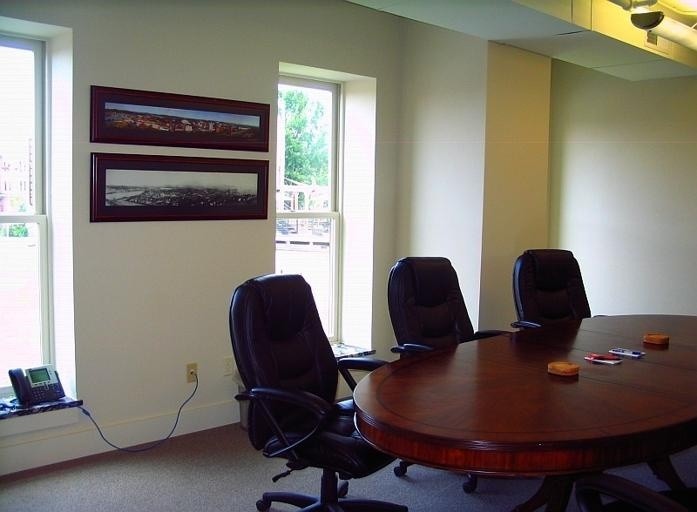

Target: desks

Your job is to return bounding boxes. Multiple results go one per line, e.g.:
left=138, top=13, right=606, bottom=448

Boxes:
left=352, top=314, right=696, bottom=511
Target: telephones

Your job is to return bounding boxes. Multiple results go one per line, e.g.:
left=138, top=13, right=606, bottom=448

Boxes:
left=9, top=363, right=66, bottom=406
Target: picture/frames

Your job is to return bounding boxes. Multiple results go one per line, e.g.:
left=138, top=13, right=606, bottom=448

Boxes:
left=89, top=154, right=269, bottom=221
left=88, top=85, right=271, bottom=153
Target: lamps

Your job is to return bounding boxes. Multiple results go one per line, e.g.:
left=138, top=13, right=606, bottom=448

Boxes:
left=628, top=0, right=697, bottom=53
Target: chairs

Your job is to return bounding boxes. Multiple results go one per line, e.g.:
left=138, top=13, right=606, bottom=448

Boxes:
left=388, top=256, right=540, bottom=492
left=574, top=467, right=697, bottom=512
left=512, top=249, right=607, bottom=329
left=228, top=273, right=410, bottom=512
